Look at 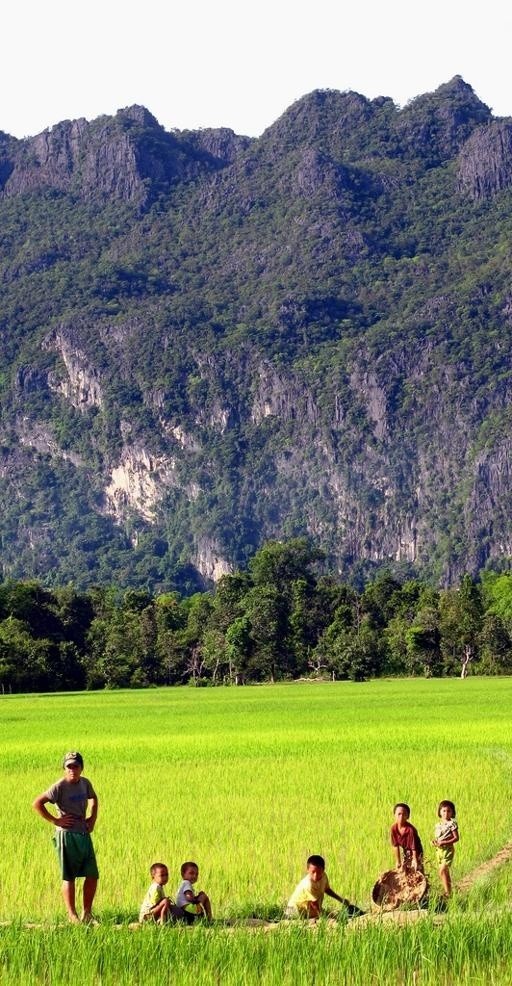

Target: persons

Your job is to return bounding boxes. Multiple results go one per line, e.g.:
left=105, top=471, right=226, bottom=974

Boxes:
left=430, top=800, right=459, bottom=898
left=390, top=803, right=430, bottom=911
left=176, top=861, right=212, bottom=923
left=32, top=751, right=99, bottom=928
left=282, top=854, right=350, bottom=920
left=139, top=862, right=171, bottom=926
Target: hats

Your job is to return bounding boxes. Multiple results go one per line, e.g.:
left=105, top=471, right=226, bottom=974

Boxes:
left=64, top=752, right=83, bottom=767
